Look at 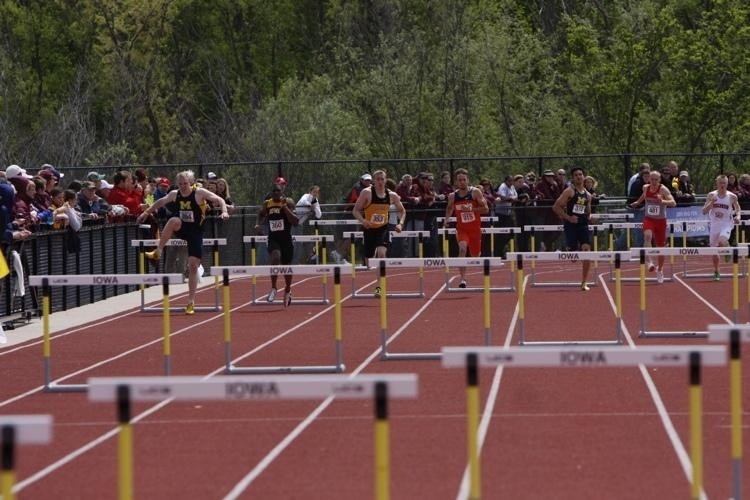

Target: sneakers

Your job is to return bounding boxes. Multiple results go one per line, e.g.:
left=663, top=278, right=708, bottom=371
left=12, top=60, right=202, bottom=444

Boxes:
left=268, top=288, right=277, bottom=302
left=582, top=281, right=589, bottom=290
left=375, top=286, right=381, bottom=296
left=186, top=303, right=194, bottom=315
left=283, top=290, right=291, bottom=307
left=725, top=251, right=731, bottom=262
left=647, top=263, right=663, bottom=284
left=713, top=270, right=720, bottom=280
left=146, top=250, right=161, bottom=262
left=459, top=280, right=467, bottom=288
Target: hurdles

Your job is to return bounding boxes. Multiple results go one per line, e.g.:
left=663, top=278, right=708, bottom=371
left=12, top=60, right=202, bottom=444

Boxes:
left=86, top=371, right=421, bottom=500
left=210, top=263, right=352, bottom=373
left=28, top=272, right=183, bottom=393
left=524, top=223, right=599, bottom=288
left=708, top=324, right=750, bottom=500
left=0, top=413, right=56, bottom=500
left=589, top=212, right=677, bottom=281
left=131, top=237, right=228, bottom=311
left=437, top=226, right=522, bottom=292
left=342, top=229, right=430, bottom=299
left=243, top=235, right=334, bottom=304
left=436, top=216, right=500, bottom=256
left=366, top=255, right=502, bottom=359
left=441, top=343, right=726, bottom=500
left=678, top=218, right=749, bottom=278
left=505, top=250, right=631, bottom=345
left=630, top=246, right=749, bottom=337
left=308, top=219, right=369, bottom=270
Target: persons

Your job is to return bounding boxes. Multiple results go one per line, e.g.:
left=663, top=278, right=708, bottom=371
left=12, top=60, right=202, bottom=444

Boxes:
left=343, top=169, right=605, bottom=266
left=136, top=170, right=229, bottom=315
left=352, top=170, right=406, bottom=297
left=626, top=160, right=696, bottom=249
left=629, top=171, right=676, bottom=283
left=702, top=172, right=750, bottom=281
left=0, top=162, right=234, bottom=243
left=445, top=169, right=489, bottom=288
left=552, top=167, right=594, bottom=292
left=256, top=174, right=323, bottom=308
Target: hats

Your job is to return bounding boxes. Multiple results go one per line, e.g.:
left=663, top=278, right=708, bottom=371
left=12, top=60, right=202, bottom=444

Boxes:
left=543, top=169, right=555, bottom=176
left=679, top=171, right=688, bottom=178
left=82, top=172, right=106, bottom=190
left=273, top=177, right=287, bottom=185
left=361, top=174, right=372, bottom=181
left=159, top=177, right=170, bottom=187
left=6, top=165, right=33, bottom=179
left=206, top=172, right=217, bottom=180
left=39, top=169, right=64, bottom=180
